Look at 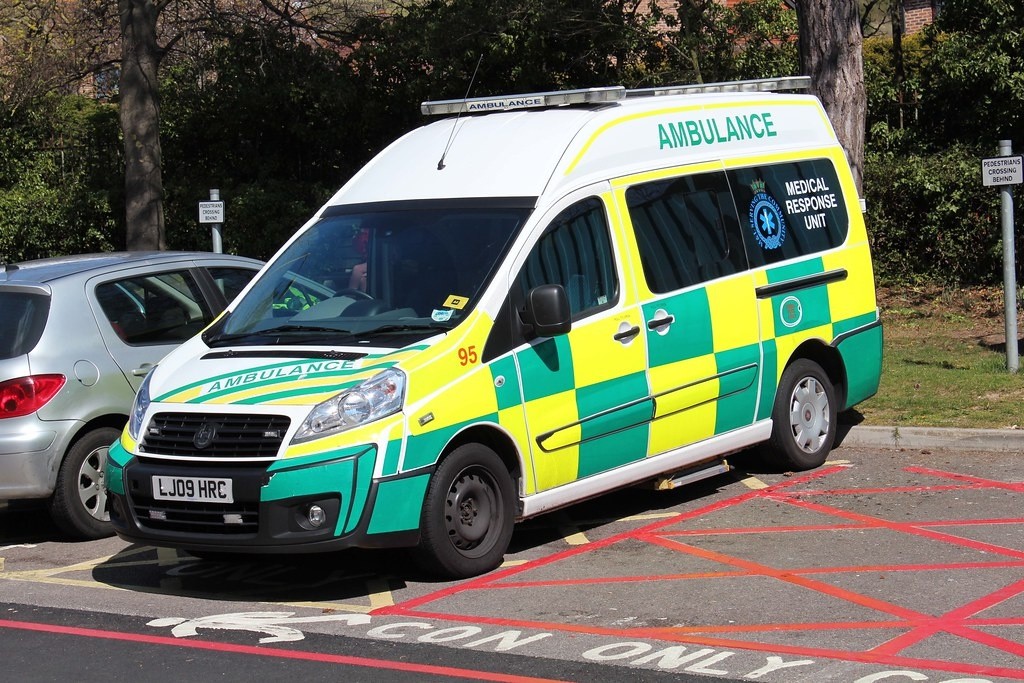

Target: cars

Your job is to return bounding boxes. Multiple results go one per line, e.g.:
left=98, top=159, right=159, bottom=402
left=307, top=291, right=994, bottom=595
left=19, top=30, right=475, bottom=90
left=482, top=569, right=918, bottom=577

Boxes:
left=0, top=250, right=350, bottom=536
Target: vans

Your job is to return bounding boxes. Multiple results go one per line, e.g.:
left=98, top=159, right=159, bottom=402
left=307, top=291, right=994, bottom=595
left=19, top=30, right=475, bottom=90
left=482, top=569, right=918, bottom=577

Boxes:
left=103, top=74, right=883, bottom=577
